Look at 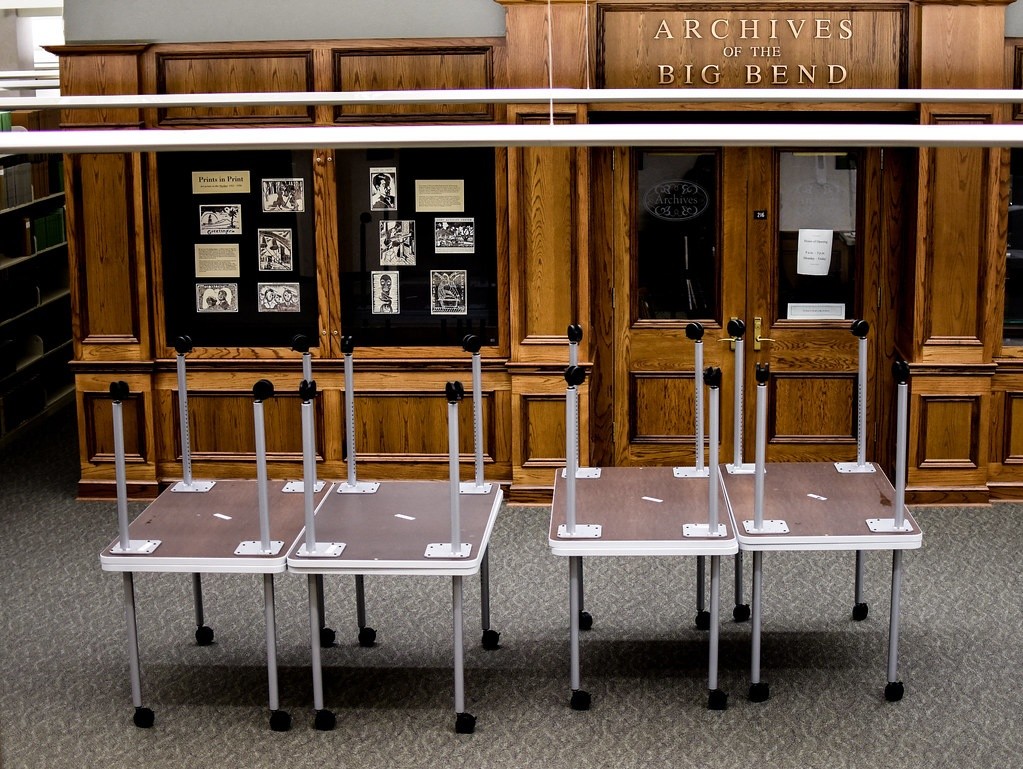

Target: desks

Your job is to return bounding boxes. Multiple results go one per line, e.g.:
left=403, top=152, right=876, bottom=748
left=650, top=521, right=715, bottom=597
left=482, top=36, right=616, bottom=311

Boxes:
left=97, top=335, right=505, bottom=734
left=547, top=318, right=925, bottom=711
